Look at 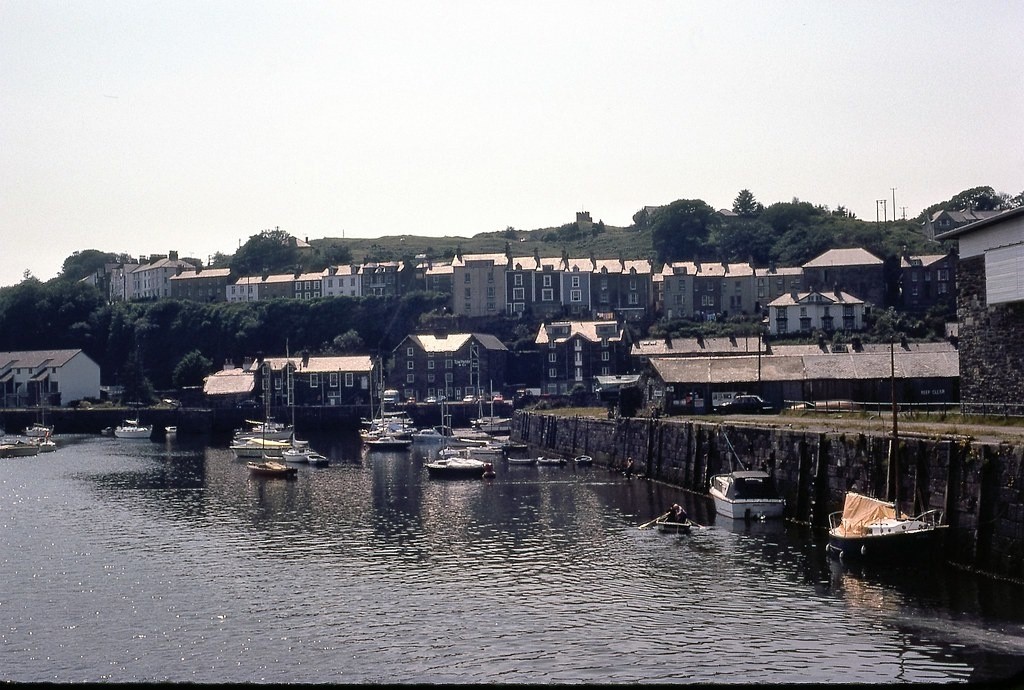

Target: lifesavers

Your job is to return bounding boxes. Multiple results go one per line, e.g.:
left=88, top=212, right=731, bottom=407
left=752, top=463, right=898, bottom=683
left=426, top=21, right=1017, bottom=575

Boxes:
left=370, top=438, right=377, bottom=441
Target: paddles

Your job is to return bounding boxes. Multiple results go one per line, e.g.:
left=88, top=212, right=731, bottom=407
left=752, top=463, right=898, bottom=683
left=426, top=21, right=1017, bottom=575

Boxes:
left=670, top=506, right=705, bottom=528
left=637, top=511, right=671, bottom=530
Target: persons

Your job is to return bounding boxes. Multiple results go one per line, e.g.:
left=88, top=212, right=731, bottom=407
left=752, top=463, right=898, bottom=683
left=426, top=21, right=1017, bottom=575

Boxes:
left=607, top=408, right=614, bottom=419
left=627, top=457, right=634, bottom=479
left=652, top=406, right=657, bottom=418
left=672, top=504, right=686, bottom=524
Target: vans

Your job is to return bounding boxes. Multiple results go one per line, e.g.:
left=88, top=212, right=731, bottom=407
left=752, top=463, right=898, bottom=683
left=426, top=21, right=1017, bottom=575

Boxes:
left=384, top=389, right=400, bottom=404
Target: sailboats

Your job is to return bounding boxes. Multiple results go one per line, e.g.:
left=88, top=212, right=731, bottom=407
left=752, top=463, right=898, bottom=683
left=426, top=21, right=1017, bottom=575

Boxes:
left=827, top=335, right=952, bottom=563
left=360, top=356, right=418, bottom=449
left=115, top=346, right=153, bottom=438
left=411, top=380, right=527, bottom=474
left=23, top=370, right=57, bottom=452
left=229, top=337, right=317, bottom=476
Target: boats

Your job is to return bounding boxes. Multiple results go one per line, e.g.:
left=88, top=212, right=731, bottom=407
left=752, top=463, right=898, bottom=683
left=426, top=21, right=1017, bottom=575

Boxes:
left=574, top=455, right=592, bottom=465
left=0, top=439, right=39, bottom=458
left=166, top=426, right=177, bottom=432
left=537, top=457, right=568, bottom=464
left=101, top=427, right=115, bottom=435
left=707, top=471, right=786, bottom=520
left=307, top=455, right=329, bottom=467
left=657, top=513, right=691, bottom=534
left=507, top=458, right=538, bottom=465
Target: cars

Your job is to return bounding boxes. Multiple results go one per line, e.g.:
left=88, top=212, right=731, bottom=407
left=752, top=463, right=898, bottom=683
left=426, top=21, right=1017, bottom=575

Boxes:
left=408, top=395, right=446, bottom=403
left=493, top=395, right=504, bottom=403
left=714, top=395, right=773, bottom=414
left=463, top=395, right=477, bottom=403
